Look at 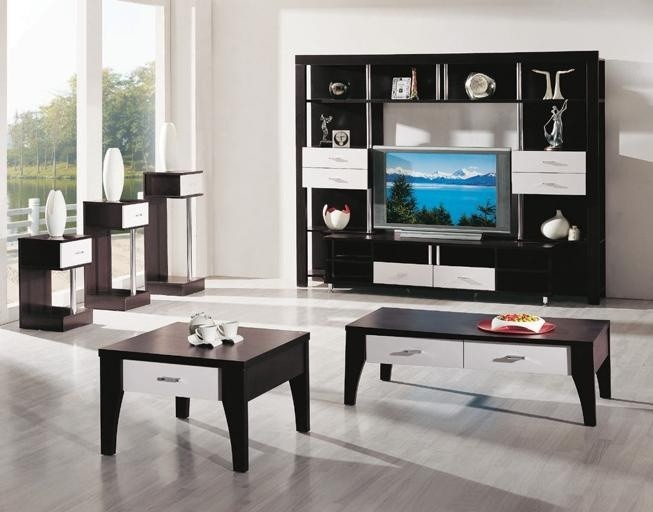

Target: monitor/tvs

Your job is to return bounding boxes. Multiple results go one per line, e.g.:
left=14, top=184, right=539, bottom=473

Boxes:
left=372, top=143, right=516, bottom=242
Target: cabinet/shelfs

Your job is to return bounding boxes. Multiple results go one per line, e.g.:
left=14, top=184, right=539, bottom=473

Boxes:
left=295, top=50, right=605, bottom=305
left=18, top=232, right=94, bottom=332
left=143, top=170, right=205, bottom=297
left=82, top=199, right=150, bottom=312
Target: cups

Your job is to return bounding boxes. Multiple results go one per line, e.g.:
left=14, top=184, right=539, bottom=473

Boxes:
left=195, top=325, right=217, bottom=339
left=216, top=321, right=240, bottom=336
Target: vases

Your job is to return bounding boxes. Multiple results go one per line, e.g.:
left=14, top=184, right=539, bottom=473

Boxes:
left=103, top=148, right=125, bottom=202
left=160, top=122, right=177, bottom=172
left=45, top=190, right=68, bottom=237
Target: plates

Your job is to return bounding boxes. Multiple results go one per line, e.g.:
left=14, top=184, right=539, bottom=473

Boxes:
left=477, top=320, right=556, bottom=335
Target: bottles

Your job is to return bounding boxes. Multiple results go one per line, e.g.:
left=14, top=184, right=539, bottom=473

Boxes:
left=567, top=226, right=581, bottom=241
